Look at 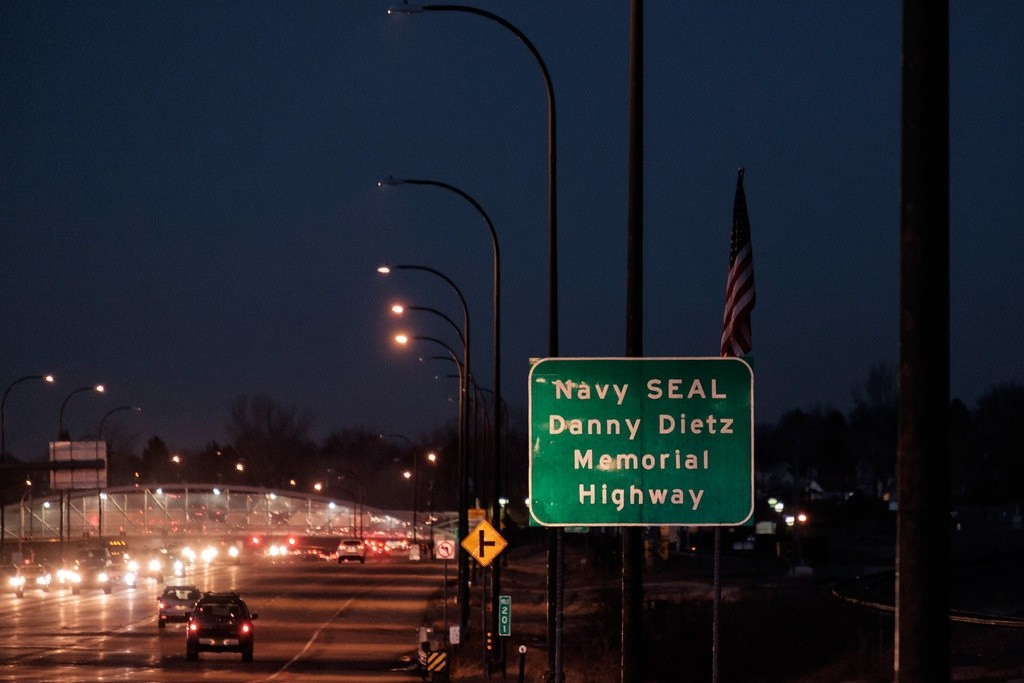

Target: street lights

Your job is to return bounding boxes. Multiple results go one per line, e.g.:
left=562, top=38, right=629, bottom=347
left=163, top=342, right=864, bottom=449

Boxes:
left=387, top=1, right=560, bottom=683
left=378, top=264, right=472, bottom=637
left=377, top=178, right=504, bottom=683
left=60, top=383, right=142, bottom=541
left=0, top=374, right=53, bottom=509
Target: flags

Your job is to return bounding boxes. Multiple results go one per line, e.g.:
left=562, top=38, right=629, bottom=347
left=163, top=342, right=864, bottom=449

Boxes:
left=720, top=185, right=758, bottom=357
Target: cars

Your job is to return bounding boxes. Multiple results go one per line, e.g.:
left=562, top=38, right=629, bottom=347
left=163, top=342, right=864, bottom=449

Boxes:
left=0, top=537, right=342, bottom=598
left=336, top=537, right=365, bottom=569
left=188, top=593, right=256, bottom=658
left=159, top=584, right=198, bottom=626
